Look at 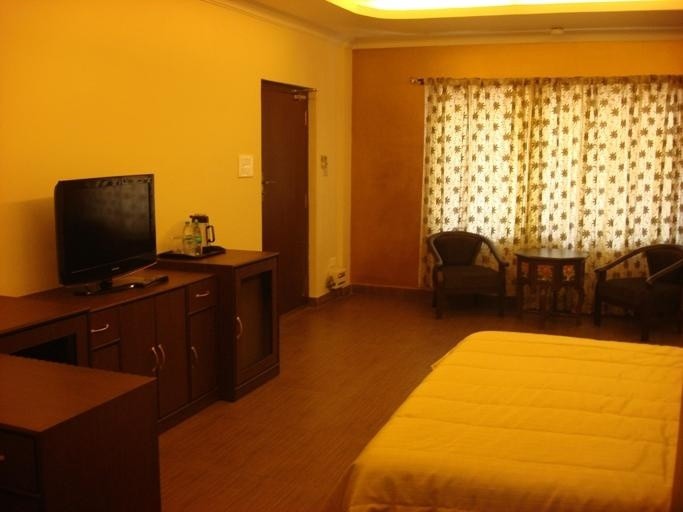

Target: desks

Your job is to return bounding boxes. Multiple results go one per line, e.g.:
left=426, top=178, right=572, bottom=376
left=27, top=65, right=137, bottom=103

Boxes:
left=2, top=352, right=160, bottom=512
left=514, top=249, right=589, bottom=329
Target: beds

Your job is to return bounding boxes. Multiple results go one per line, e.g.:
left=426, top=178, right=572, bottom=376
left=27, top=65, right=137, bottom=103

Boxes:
left=322, top=331, right=683, bottom=512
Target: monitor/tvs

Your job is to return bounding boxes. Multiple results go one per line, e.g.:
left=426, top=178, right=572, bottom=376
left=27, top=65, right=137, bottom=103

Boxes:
left=53, top=173, right=156, bottom=295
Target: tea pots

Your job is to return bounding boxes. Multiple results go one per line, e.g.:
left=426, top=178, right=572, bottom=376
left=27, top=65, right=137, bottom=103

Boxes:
left=190, top=215, right=215, bottom=253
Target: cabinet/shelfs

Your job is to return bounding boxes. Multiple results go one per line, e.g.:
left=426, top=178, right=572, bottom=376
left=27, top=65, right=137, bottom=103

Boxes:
left=186, top=276, right=223, bottom=400
left=233, top=256, right=280, bottom=386
left=120, top=287, right=189, bottom=421
left=89, top=305, right=120, bottom=373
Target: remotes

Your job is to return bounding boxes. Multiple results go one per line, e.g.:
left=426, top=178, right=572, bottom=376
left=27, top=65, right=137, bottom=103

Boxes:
left=145, top=273, right=169, bottom=285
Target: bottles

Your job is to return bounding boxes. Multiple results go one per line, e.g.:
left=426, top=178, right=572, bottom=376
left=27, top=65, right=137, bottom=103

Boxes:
left=182, top=221, right=193, bottom=256
left=192, top=219, right=202, bottom=255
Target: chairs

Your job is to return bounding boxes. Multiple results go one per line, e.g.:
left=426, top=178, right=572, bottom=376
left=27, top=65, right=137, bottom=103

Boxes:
left=595, top=244, right=683, bottom=342
left=427, top=232, right=510, bottom=320
